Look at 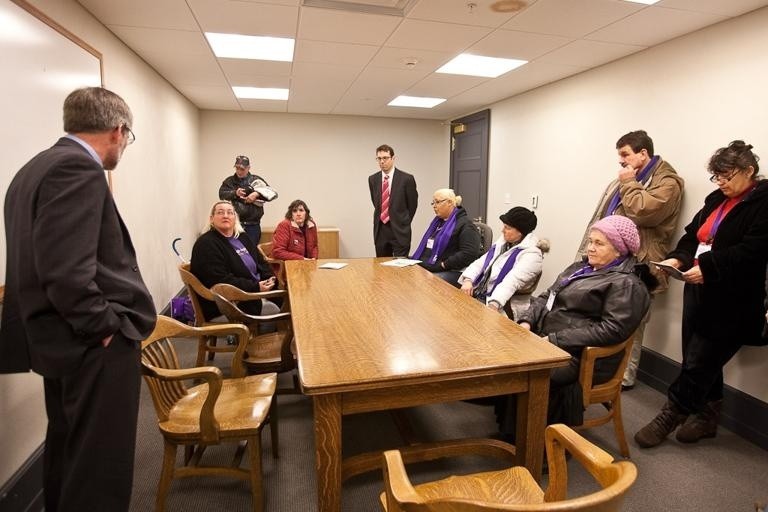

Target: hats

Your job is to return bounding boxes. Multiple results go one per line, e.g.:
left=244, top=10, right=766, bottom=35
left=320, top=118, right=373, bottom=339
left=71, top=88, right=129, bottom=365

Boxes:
left=590, top=214, right=641, bottom=258
left=499, top=206, right=538, bottom=235
left=234, top=155, right=250, bottom=169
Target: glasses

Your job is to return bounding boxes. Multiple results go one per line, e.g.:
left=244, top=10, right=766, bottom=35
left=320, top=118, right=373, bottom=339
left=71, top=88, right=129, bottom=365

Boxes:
left=124, top=125, right=135, bottom=146
left=431, top=198, right=449, bottom=206
left=378, top=156, right=391, bottom=163
left=709, top=169, right=744, bottom=184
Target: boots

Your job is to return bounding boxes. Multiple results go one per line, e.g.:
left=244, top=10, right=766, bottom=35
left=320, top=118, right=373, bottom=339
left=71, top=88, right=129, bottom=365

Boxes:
left=675, top=401, right=721, bottom=443
left=635, top=399, right=690, bottom=448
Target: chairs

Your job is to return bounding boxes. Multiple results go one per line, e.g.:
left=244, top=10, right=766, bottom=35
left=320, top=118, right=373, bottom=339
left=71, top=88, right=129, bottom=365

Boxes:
left=178, top=263, right=257, bottom=383
left=210, top=281, right=302, bottom=398
left=255, top=240, right=301, bottom=291
left=499, top=269, right=544, bottom=323
left=567, top=327, right=639, bottom=460
left=467, top=218, right=494, bottom=266
left=378, top=423, right=639, bottom=512
left=134, top=313, right=282, bottom=512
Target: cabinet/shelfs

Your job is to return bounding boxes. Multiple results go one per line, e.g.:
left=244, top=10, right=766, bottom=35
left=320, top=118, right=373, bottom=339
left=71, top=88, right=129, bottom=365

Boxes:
left=258, top=228, right=343, bottom=261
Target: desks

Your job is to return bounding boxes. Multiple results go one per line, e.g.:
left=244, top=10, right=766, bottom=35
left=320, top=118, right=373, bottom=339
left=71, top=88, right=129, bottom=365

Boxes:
left=285, top=257, right=573, bottom=509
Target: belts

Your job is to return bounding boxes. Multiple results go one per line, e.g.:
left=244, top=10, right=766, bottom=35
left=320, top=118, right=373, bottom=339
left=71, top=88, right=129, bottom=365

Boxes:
left=240, top=220, right=260, bottom=225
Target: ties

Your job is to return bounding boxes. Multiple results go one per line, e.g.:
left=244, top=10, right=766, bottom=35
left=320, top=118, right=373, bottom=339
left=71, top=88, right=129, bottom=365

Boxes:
left=379, top=175, right=391, bottom=225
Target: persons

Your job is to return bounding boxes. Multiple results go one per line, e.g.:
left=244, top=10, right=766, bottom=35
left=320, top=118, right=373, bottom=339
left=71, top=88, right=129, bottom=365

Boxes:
left=576, top=131, right=685, bottom=393
left=408, top=189, right=485, bottom=288
left=219, top=156, right=275, bottom=246
left=634, top=139, right=768, bottom=449
left=495, top=215, right=651, bottom=474
left=0, top=87, right=157, bottom=512
left=191, top=200, right=282, bottom=333
left=368, top=145, right=418, bottom=256
left=457, top=207, right=550, bottom=313
left=272, top=200, right=319, bottom=260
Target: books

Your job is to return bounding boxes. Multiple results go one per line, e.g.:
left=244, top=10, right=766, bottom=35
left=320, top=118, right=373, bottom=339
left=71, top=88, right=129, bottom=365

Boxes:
left=318, top=262, right=347, bottom=271
left=649, top=260, right=686, bottom=283
